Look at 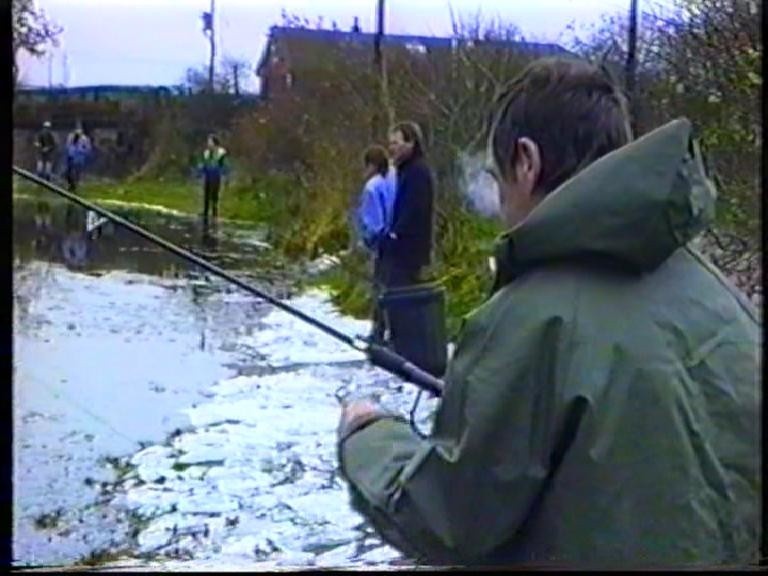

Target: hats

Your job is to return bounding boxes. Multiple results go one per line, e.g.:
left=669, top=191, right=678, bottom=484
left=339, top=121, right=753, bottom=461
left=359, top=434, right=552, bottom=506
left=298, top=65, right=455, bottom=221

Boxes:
left=43, top=122, right=52, bottom=128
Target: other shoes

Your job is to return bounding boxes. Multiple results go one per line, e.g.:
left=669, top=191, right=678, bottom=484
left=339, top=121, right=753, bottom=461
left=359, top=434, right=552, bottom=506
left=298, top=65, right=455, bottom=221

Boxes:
left=356, top=334, right=369, bottom=342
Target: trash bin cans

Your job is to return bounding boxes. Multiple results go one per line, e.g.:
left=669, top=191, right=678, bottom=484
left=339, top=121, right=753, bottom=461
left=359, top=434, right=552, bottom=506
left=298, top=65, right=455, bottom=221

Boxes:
left=378, top=279, right=448, bottom=380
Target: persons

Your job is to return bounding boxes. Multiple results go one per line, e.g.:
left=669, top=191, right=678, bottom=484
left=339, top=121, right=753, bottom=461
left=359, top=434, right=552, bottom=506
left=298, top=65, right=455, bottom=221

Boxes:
left=34, top=121, right=92, bottom=195
left=201, top=134, right=231, bottom=233
left=355, top=121, right=434, bottom=343
left=336, top=57, right=764, bottom=572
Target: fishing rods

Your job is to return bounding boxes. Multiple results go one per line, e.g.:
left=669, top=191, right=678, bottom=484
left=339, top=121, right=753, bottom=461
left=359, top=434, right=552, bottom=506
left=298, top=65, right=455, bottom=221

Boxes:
left=12, top=163, right=446, bottom=401
